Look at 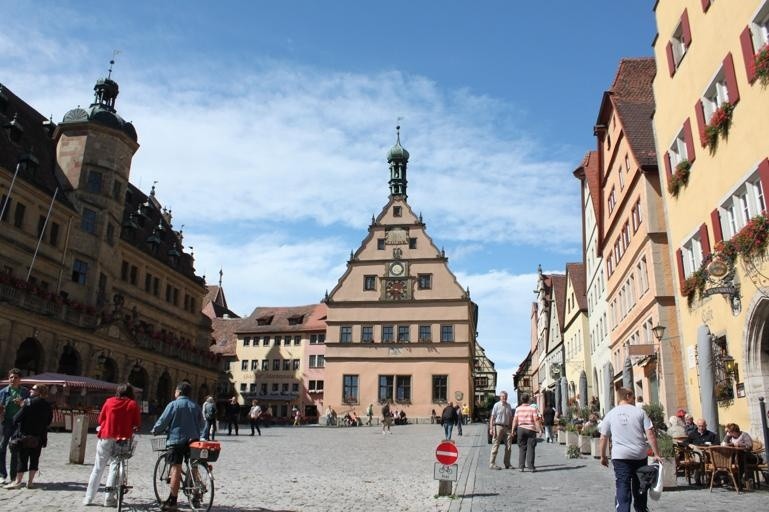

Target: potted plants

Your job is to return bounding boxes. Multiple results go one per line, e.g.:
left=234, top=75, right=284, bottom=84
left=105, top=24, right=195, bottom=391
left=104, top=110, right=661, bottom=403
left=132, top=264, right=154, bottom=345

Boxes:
left=557, top=394, right=676, bottom=491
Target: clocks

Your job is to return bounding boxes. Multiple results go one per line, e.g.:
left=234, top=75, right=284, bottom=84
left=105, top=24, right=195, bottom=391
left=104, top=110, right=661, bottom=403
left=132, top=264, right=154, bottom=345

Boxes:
left=386, top=280, right=407, bottom=301
left=391, top=265, right=403, bottom=275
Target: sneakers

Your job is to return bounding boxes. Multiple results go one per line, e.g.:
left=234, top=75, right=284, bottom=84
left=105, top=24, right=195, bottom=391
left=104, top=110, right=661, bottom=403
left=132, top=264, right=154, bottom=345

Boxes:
left=490, top=465, right=501, bottom=470
left=161, top=500, right=177, bottom=511
left=1, top=476, right=33, bottom=489
left=505, top=464, right=514, bottom=468
left=104, top=495, right=116, bottom=506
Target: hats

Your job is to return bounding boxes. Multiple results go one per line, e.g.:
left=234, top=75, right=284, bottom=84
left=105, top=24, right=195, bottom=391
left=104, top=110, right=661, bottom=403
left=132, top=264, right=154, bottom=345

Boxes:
left=677, top=409, right=686, bottom=416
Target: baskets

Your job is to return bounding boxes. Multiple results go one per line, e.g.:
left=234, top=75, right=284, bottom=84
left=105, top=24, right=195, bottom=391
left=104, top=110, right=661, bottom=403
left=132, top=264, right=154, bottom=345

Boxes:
left=111, top=440, right=138, bottom=458
left=151, top=437, right=169, bottom=451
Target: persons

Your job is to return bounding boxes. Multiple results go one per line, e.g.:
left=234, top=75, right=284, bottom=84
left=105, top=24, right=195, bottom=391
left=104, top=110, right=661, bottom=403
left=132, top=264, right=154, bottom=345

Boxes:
left=2, top=383, right=53, bottom=489
left=488, top=391, right=515, bottom=470
left=511, top=394, right=543, bottom=473
left=598, top=387, right=664, bottom=512
left=325, top=398, right=407, bottom=435
left=0, top=368, right=30, bottom=485
left=530, top=396, right=559, bottom=443
left=82, top=382, right=142, bottom=507
left=201, top=396, right=301, bottom=441
left=666, top=409, right=757, bottom=489
left=150, top=381, right=205, bottom=509
left=432, top=401, right=480, bottom=441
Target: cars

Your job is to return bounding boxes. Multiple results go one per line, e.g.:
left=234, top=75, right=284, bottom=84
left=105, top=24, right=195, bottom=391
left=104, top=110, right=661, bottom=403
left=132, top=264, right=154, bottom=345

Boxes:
left=484, top=409, right=517, bottom=445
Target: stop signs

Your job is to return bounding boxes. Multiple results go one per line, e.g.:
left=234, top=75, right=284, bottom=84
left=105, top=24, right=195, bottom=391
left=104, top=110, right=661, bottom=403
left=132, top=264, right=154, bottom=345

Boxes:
left=435, top=442, right=458, bottom=465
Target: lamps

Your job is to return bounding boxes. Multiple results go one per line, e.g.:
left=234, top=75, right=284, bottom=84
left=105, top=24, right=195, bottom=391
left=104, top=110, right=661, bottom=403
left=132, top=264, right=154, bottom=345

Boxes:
left=718, top=351, right=739, bottom=383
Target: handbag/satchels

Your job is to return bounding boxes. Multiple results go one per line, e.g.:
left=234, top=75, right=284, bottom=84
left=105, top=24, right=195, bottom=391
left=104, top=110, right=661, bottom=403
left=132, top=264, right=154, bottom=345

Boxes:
left=636, top=461, right=659, bottom=495
left=9, top=431, right=38, bottom=449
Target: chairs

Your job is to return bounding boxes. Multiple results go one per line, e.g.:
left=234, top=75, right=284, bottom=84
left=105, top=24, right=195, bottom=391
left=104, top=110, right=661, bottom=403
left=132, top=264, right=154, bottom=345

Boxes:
left=673, top=436, right=769, bottom=495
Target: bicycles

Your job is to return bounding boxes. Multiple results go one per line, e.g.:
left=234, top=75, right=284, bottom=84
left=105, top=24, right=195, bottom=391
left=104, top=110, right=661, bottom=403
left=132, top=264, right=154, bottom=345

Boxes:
left=103, top=426, right=142, bottom=512
left=149, top=430, right=220, bottom=512
